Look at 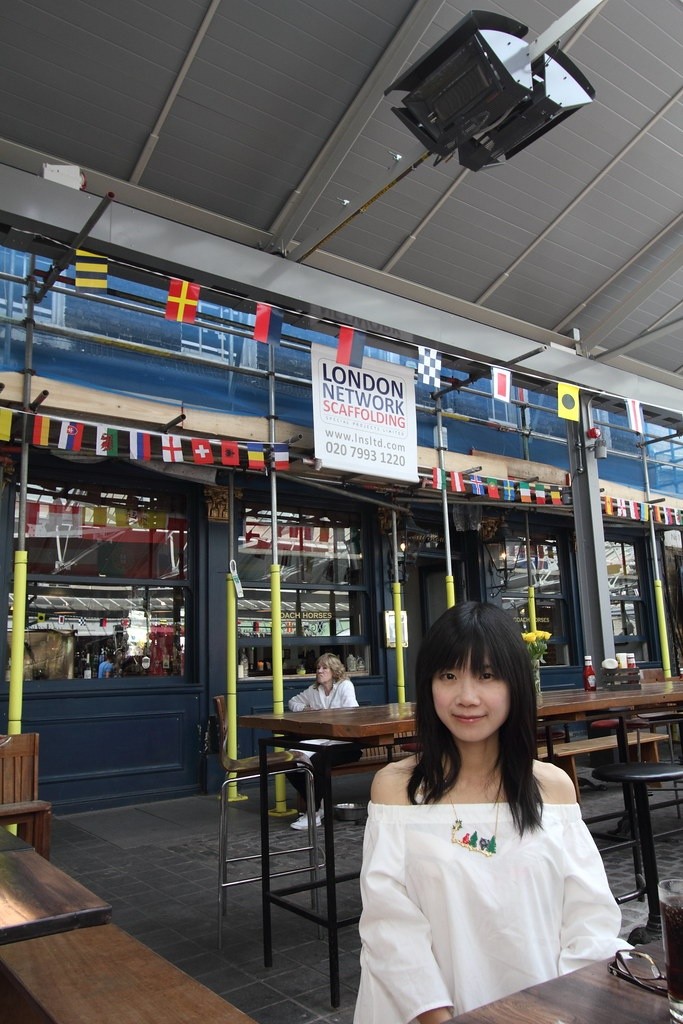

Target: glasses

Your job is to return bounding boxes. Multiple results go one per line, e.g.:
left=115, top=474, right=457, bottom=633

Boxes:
left=607, top=949, right=668, bottom=998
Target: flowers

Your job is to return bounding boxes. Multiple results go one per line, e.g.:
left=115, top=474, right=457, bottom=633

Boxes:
left=522, top=631, right=553, bottom=693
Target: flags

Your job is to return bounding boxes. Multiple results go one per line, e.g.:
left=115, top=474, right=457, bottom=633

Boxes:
left=603, top=497, right=683, bottom=525
left=415, top=346, right=443, bottom=388
left=24, top=501, right=363, bottom=553
left=623, top=399, right=648, bottom=433
left=430, top=469, right=563, bottom=507
left=494, top=537, right=561, bottom=558
left=337, top=328, right=367, bottom=367
left=1, top=408, right=291, bottom=473
left=491, top=366, right=514, bottom=405
left=555, top=385, right=584, bottom=423
left=73, top=247, right=110, bottom=298
left=251, top=303, right=284, bottom=346
left=161, top=280, right=202, bottom=323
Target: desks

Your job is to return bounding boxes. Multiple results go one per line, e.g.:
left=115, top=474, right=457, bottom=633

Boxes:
left=0, top=923, right=260, bottom=1024
left=0, top=826, right=114, bottom=943
left=445, top=942, right=670, bottom=1024
left=239, top=679, right=683, bottom=1006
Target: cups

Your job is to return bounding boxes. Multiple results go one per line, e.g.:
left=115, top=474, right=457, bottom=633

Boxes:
left=297, top=665, right=305, bottom=674
left=238, top=665, right=243, bottom=678
left=657, top=880, right=683, bottom=1023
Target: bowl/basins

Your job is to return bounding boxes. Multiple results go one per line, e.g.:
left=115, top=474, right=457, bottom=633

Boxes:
left=335, top=807, right=365, bottom=820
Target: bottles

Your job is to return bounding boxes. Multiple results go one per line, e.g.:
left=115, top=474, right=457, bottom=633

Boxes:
left=84, top=653, right=91, bottom=680
left=584, top=656, right=596, bottom=691
left=627, top=653, right=637, bottom=684
left=347, top=654, right=356, bottom=672
left=240, top=653, right=248, bottom=677
left=5, top=657, right=11, bottom=681
left=356, top=656, right=365, bottom=670
left=141, top=643, right=150, bottom=675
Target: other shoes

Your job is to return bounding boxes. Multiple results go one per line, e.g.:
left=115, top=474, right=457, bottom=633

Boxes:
left=290, top=812, right=321, bottom=830
left=316, top=798, right=324, bottom=820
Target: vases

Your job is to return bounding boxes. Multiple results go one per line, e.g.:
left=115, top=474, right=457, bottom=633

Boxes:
left=528, top=659, right=542, bottom=701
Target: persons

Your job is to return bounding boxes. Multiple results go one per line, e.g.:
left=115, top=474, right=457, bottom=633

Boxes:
left=98, top=651, right=119, bottom=678
left=280, top=651, right=364, bottom=830
left=350, top=601, right=636, bottom=1024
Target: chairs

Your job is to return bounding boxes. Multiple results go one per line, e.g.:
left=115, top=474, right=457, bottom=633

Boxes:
left=213, top=694, right=326, bottom=913
left=0, top=733, right=52, bottom=862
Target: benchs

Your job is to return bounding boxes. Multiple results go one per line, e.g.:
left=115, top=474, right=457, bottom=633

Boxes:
left=330, top=748, right=412, bottom=780
left=538, top=732, right=668, bottom=803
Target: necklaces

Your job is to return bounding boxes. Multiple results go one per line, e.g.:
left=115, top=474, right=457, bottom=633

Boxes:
left=436, top=769, right=506, bottom=859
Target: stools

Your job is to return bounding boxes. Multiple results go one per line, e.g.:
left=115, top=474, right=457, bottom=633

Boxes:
left=586, top=719, right=622, bottom=762
left=593, top=763, right=683, bottom=934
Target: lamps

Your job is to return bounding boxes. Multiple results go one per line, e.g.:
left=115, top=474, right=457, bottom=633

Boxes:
left=588, top=440, right=608, bottom=460
